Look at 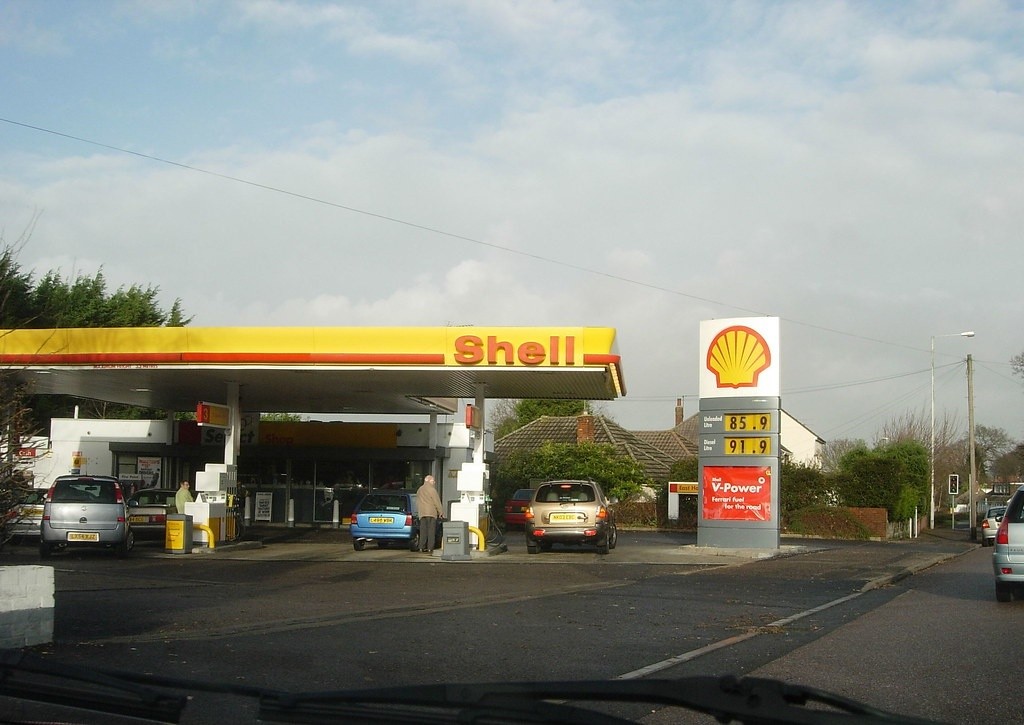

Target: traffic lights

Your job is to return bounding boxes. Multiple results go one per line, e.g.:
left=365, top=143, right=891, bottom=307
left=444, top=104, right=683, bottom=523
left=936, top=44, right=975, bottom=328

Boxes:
left=949, top=475, right=958, bottom=494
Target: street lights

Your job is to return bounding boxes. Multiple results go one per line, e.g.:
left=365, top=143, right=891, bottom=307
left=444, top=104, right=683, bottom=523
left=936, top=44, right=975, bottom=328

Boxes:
left=930, top=331, right=976, bottom=531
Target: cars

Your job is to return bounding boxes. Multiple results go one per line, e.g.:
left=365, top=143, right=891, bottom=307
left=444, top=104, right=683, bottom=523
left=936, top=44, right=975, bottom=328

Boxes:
left=39, top=474, right=133, bottom=559
left=125, top=488, right=177, bottom=541
left=503, top=489, right=535, bottom=531
left=991, top=483, right=1024, bottom=603
left=6, top=488, right=50, bottom=546
left=350, top=492, right=444, bottom=552
left=981, top=505, right=1009, bottom=547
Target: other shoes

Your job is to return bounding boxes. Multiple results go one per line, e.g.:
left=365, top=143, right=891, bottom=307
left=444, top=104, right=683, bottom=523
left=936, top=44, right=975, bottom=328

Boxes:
left=420, top=548, right=432, bottom=552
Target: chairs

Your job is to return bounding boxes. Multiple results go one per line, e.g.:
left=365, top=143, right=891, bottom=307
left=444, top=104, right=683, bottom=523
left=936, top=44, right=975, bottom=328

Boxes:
left=571, top=491, right=580, bottom=501
left=578, top=493, right=587, bottom=502
left=545, top=493, right=559, bottom=501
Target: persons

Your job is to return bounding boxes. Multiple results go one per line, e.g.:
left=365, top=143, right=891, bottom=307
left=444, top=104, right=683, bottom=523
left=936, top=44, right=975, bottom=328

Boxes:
left=176, top=480, right=193, bottom=515
left=237, top=482, right=246, bottom=508
left=416, top=475, right=444, bottom=553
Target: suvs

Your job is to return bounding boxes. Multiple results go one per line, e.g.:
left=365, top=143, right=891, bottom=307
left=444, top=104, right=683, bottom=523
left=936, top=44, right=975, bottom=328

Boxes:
left=525, top=478, right=617, bottom=554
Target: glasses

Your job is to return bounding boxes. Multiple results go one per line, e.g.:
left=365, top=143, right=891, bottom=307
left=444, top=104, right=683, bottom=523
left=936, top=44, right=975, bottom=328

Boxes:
left=184, top=484, right=189, bottom=486
